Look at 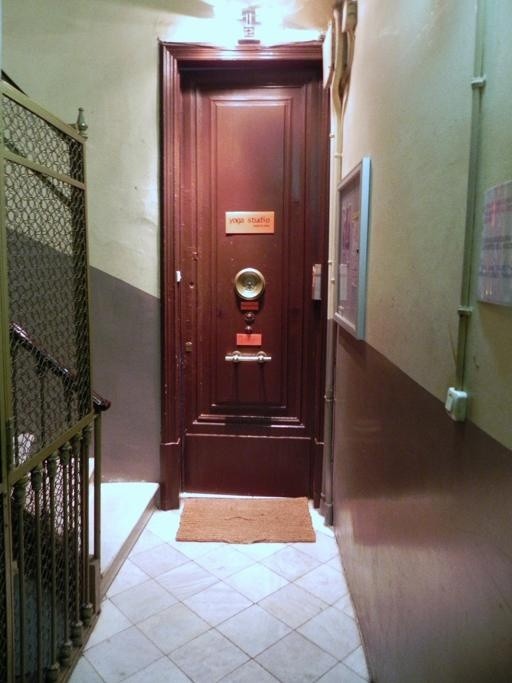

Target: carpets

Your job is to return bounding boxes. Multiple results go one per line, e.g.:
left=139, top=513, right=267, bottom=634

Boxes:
left=176, top=497, right=317, bottom=543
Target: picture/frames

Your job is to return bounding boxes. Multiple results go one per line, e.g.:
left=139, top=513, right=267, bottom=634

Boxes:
left=332, top=156, right=370, bottom=340
